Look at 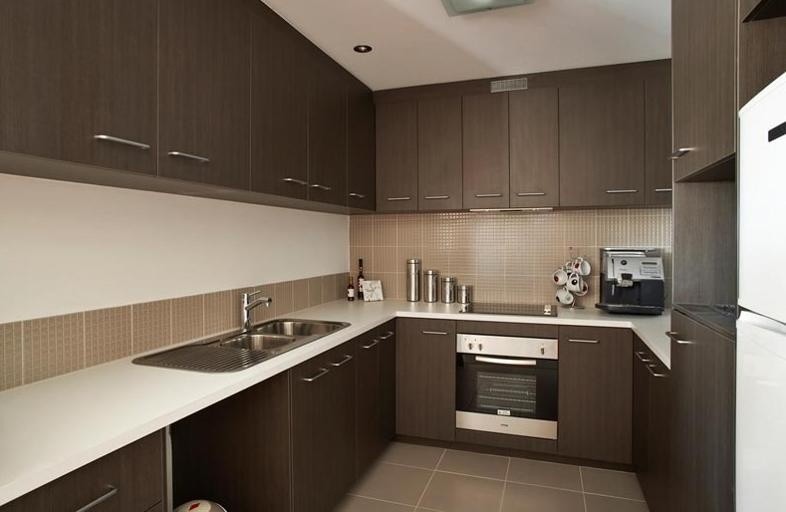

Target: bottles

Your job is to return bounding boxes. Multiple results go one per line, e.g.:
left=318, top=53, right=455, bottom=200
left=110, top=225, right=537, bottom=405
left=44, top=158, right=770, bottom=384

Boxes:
left=357, top=258, right=366, bottom=300
left=347, top=277, right=354, bottom=301
left=456, top=284, right=472, bottom=304
left=423, top=269, right=439, bottom=302
left=407, top=257, right=420, bottom=301
left=440, top=277, right=456, bottom=302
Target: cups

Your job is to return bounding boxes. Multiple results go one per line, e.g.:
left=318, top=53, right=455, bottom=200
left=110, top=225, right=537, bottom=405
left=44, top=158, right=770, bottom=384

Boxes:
left=552, top=257, right=591, bottom=305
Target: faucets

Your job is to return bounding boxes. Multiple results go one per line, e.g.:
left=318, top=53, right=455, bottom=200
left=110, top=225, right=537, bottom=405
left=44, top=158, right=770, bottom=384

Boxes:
left=241, top=289, right=272, bottom=333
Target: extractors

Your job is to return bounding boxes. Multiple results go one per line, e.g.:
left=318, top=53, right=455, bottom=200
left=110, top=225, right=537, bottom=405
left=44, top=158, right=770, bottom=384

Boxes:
left=469, top=207, right=554, bottom=215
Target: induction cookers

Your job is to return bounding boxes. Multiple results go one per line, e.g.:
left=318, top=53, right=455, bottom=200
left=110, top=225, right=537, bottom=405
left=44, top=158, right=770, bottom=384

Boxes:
left=458, top=302, right=559, bottom=318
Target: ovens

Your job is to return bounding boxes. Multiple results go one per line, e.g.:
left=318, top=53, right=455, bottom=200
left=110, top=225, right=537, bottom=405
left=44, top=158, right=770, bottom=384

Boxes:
left=454, top=332, right=558, bottom=440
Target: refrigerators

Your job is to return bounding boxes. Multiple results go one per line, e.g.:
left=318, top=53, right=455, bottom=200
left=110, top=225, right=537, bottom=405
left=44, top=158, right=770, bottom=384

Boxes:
left=736, top=71, right=786, bottom=511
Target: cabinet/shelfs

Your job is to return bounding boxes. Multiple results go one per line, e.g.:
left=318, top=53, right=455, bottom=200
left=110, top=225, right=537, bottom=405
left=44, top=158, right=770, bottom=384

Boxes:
left=355, top=318, right=396, bottom=483
left=670, top=0, right=738, bottom=188
left=632, top=329, right=670, bottom=512
left=346, top=73, right=375, bottom=216
left=667, top=310, right=737, bottom=512
left=188, top=340, right=356, bottom=510
left=461, top=87, right=560, bottom=213
left=0, top=0, right=252, bottom=201
left=557, top=323, right=634, bottom=466
left=0, top=426, right=166, bottom=511
left=558, top=76, right=671, bottom=216
left=372, top=96, right=462, bottom=212
left=394, top=316, right=458, bottom=442
left=253, top=0, right=349, bottom=215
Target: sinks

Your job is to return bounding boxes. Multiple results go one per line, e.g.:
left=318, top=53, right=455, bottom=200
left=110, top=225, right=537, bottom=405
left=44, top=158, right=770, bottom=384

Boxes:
left=218, top=319, right=351, bottom=354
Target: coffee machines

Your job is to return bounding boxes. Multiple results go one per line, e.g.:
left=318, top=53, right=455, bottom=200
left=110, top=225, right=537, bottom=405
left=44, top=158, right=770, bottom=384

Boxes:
left=594, top=246, right=666, bottom=315
